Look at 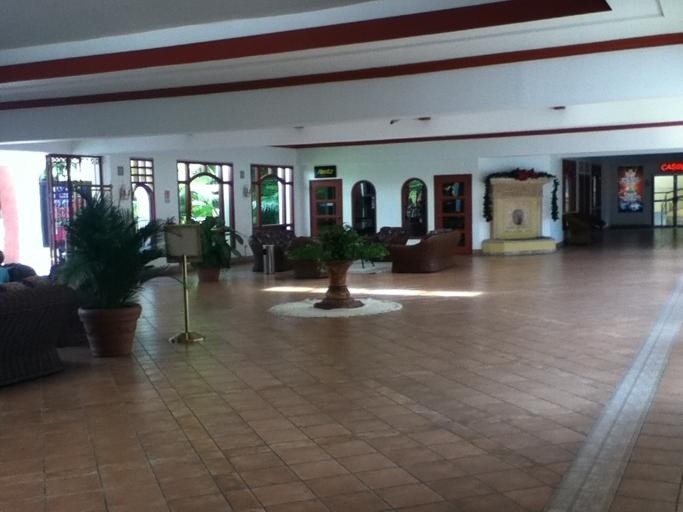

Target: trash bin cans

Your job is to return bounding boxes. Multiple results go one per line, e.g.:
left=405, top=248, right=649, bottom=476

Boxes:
left=262, top=244, right=276, bottom=274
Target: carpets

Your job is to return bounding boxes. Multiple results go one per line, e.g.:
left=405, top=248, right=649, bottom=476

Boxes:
left=346, top=259, right=392, bottom=273
left=267, top=299, right=403, bottom=317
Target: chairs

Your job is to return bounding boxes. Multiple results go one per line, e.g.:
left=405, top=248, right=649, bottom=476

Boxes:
left=0, top=264, right=87, bottom=387
left=359, top=227, right=460, bottom=273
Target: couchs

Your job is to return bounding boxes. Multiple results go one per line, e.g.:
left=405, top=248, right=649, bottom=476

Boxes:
left=250, top=224, right=311, bottom=273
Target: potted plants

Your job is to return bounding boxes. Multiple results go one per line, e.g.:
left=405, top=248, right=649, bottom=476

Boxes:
left=182, top=215, right=244, bottom=283
left=51, top=182, right=193, bottom=358
left=285, top=224, right=391, bottom=309
